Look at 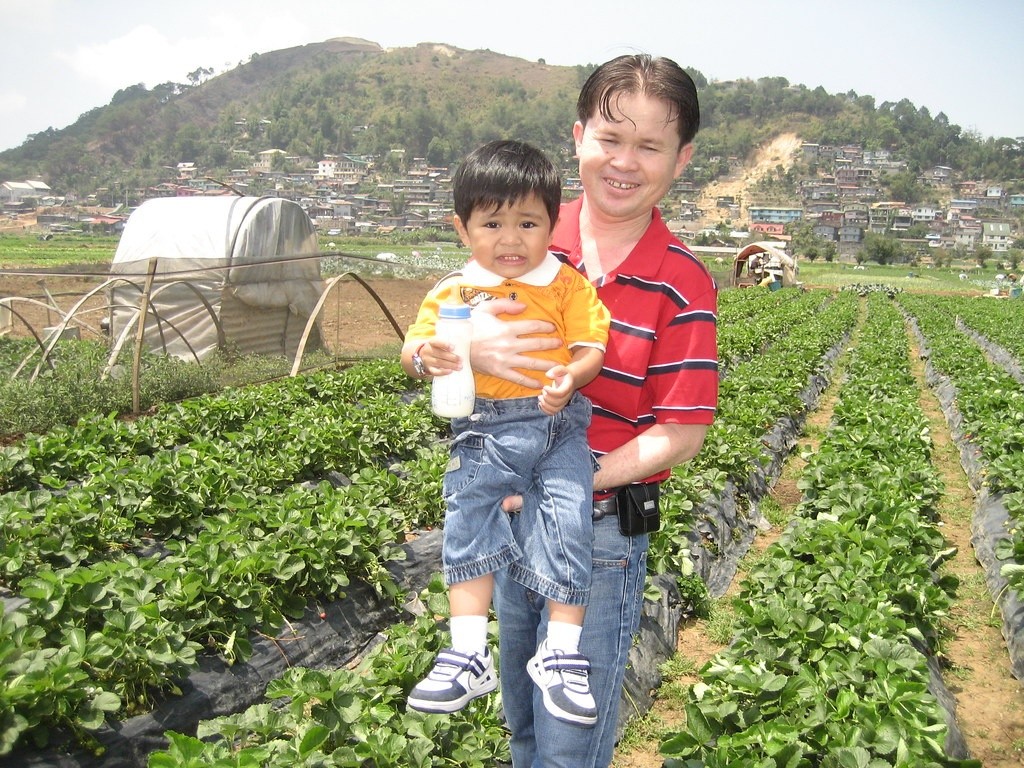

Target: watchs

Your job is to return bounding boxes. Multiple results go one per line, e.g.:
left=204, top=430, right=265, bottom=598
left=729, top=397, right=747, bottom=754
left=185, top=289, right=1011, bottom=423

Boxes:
left=412, top=343, right=430, bottom=376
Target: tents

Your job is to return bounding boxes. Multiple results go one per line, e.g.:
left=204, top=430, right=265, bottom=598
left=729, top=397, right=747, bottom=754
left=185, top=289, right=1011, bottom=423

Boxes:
left=108, top=196, right=322, bottom=362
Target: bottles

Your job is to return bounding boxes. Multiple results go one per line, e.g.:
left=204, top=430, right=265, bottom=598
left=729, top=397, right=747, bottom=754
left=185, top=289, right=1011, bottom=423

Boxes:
left=430, top=282, right=475, bottom=418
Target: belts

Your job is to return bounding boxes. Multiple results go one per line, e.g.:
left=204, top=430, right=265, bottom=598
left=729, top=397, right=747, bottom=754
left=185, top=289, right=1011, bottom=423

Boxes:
left=593, top=498, right=616, bottom=521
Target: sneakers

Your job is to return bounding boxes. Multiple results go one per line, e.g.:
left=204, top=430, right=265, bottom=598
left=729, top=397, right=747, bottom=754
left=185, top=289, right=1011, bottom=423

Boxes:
left=526, top=639, right=599, bottom=728
left=408, top=642, right=500, bottom=714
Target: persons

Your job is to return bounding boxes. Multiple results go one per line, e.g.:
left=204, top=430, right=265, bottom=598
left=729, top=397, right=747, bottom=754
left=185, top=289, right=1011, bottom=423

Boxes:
left=995, top=274, right=1006, bottom=282
left=1007, top=273, right=1017, bottom=282
left=750, top=253, right=763, bottom=275
left=959, top=273, right=969, bottom=280
left=402, top=140, right=610, bottom=728
left=468, top=54, right=720, bottom=768
